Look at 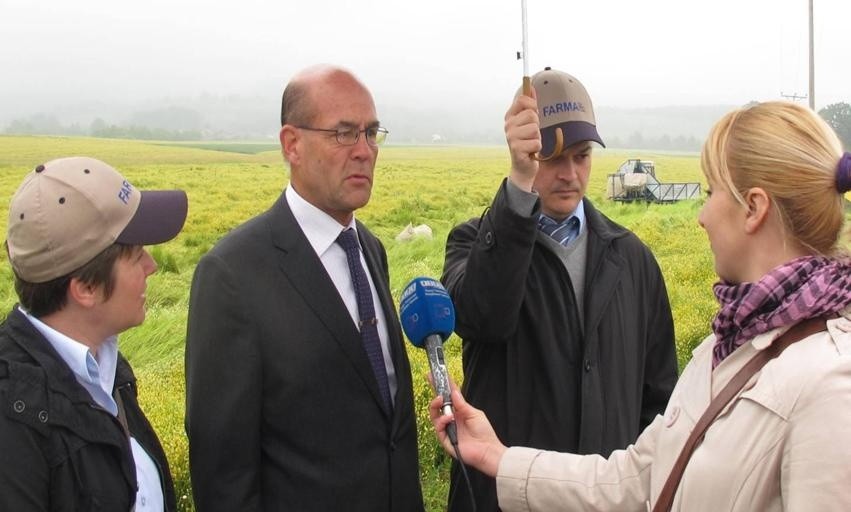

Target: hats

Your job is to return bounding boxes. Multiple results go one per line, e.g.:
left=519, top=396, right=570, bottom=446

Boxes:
left=513, top=67, right=605, bottom=157
left=6, top=156, right=188, bottom=284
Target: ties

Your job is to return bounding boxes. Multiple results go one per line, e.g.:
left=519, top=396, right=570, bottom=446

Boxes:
left=537, top=217, right=579, bottom=247
left=335, top=227, right=393, bottom=425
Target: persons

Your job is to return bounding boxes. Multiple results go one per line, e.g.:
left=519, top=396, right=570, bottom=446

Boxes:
left=1, top=157, right=189, bottom=512
left=439, top=66, right=679, bottom=512
left=183, top=65, right=426, bottom=510
left=427, top=100, right=851, bottom=512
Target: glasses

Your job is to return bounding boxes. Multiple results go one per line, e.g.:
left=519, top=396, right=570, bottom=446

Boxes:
left=295, top=125, right=388, bottom=147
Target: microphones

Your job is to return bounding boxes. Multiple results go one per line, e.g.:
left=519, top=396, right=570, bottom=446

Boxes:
left=399, top=277, right=458, bottom=444
